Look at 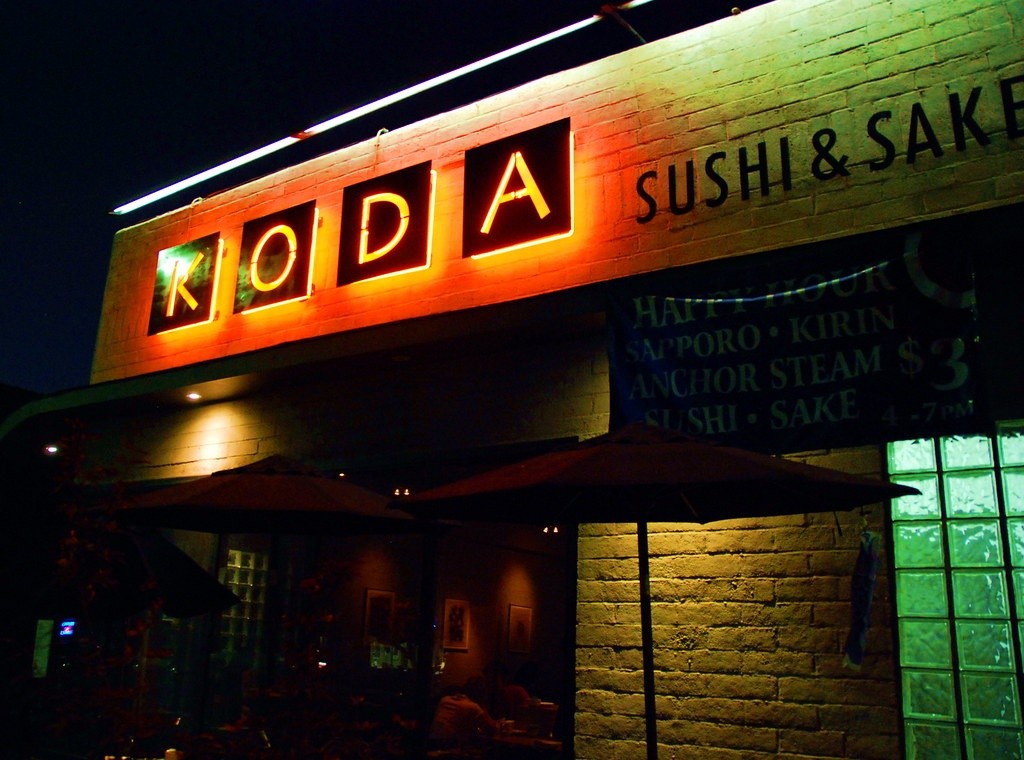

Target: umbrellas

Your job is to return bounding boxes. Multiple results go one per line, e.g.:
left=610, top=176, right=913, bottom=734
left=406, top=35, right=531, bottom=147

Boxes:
left=106, top=455, right=462, bottom=756
left=390, top=419, right=922, bottom=760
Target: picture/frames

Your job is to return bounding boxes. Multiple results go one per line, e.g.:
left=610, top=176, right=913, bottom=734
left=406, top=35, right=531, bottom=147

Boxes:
left=365, top=588, right=535, bottom=656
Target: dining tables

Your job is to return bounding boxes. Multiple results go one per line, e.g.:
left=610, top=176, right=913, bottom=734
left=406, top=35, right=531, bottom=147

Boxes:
left=468, top=700, right=568, bottom=759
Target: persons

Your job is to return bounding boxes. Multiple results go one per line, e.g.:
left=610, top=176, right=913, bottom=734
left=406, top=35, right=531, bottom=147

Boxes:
left=480, top=659, right=532, bottom=722
left=428, top=682, right=496, bottom=758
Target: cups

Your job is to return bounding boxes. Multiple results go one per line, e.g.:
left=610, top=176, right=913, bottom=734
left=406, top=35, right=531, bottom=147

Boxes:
left=501, top=720, right=515, bottom=734
